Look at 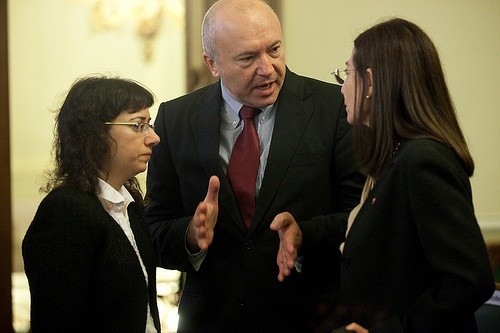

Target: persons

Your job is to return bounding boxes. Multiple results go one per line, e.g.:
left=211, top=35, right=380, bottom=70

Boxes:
left=22, top=76, right=159, bottom=333
left=321, top=18, right=495, bottom=333
left=142, top=0, right=368, bottom=333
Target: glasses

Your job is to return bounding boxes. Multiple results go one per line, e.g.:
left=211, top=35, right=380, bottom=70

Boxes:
left=103, top=121, right=153, bottom=133
left=333, top=68, right=361, bottom=83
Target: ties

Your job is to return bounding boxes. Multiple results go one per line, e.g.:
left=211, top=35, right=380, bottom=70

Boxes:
left=226, top=105, right=263, bottom=230
